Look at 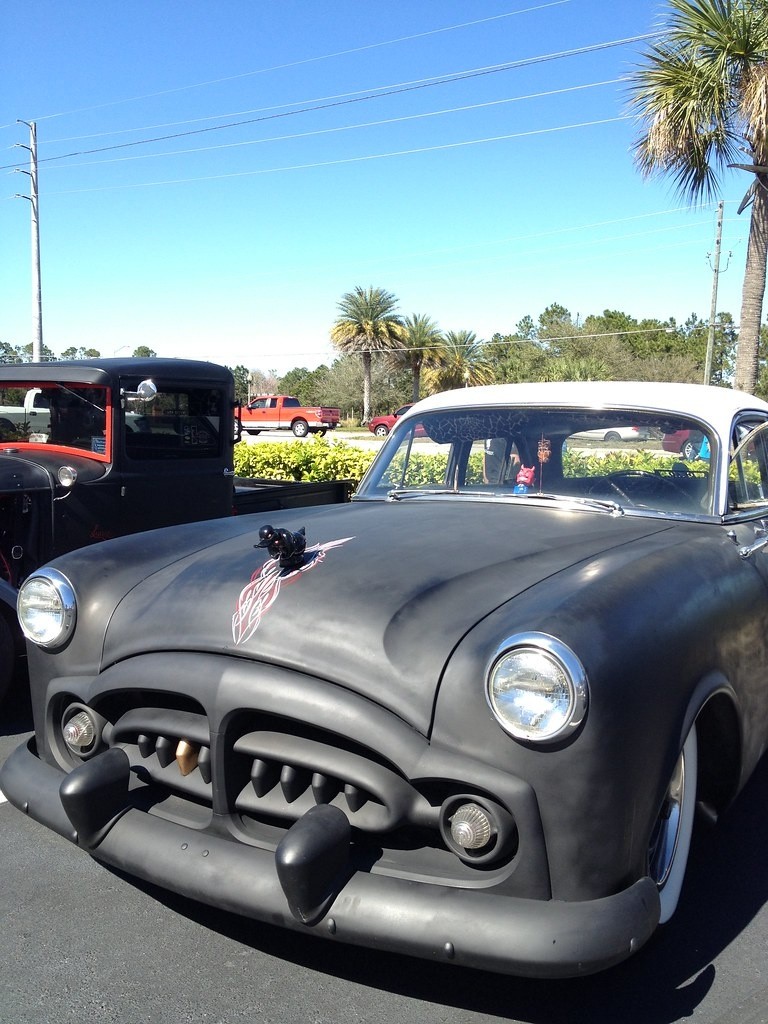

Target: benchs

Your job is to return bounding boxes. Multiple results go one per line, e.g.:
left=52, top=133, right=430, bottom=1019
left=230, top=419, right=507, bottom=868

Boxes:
left=592, top=474, right=761, bottom=501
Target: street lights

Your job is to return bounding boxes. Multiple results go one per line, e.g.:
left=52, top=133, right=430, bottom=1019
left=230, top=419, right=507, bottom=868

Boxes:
left=246, top=375, right=253, bottom=404
left=464, top=372, right=470, bottom=387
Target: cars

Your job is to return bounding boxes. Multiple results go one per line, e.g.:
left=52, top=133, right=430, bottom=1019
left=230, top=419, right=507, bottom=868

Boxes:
left=369, top=403, right=429, bottom=438
left=568, top=426, right=648, bottom=442
left=662, top=429, right=757, bottom=461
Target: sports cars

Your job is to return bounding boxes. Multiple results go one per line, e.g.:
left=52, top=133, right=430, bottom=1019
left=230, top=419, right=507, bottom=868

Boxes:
left=0, top=380, right=768, bottom=979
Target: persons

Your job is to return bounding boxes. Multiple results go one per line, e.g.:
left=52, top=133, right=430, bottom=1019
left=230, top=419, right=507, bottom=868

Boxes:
left=687, top=429, right=711, bottom=462
left=482, top=437, right=520, bottom=483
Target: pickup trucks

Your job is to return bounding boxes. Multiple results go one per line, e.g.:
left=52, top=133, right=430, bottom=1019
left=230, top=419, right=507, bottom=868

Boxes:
left=233, top=395, right=341, bottom=437
left=0, top=357, right=359, bottom=706
